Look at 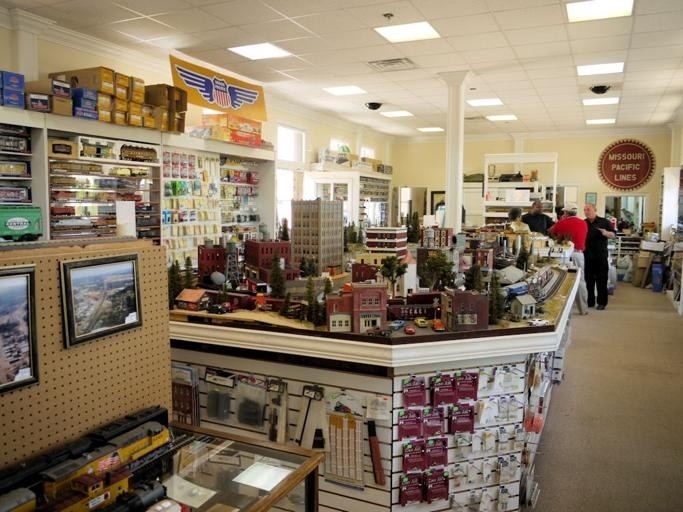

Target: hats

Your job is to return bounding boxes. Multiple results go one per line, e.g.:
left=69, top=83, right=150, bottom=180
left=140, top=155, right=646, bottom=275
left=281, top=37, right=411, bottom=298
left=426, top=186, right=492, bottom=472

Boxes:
left=562, top=203, right=577, bottom=211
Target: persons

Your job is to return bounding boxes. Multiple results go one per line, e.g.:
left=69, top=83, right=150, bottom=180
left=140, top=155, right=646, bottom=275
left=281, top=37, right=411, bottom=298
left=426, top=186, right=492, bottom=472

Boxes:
left=508, top=206, right=532, bottom=235
left=520, top=200, right=555, bottom=236
left=582, top=201, right=616, bottom=310
left=547, top=202, right=589, bottom=315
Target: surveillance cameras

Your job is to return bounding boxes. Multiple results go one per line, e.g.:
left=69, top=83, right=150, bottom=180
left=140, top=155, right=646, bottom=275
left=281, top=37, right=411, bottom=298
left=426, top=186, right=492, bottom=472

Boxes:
left=364, top=102, right=382, bottom=111
left=590, top=84, right=610, bottom=94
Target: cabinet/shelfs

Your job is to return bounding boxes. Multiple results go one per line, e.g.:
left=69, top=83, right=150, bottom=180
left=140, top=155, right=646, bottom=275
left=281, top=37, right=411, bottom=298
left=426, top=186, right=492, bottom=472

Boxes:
left=0, top=106, right=162, bottom=241
left=482, top=153, right=558, bottom=226
left=147, top=418, right=326, bottom=511
left=162, top=131, right=277, bottom=277
left=306, top=170, right=393, bottom=229
left=607, top=236, right=644, bottom=277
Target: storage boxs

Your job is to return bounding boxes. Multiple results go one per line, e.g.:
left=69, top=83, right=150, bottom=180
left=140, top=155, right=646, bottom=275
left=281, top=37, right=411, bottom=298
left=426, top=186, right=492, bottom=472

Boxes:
left=0, top=66, right=263, bottom=148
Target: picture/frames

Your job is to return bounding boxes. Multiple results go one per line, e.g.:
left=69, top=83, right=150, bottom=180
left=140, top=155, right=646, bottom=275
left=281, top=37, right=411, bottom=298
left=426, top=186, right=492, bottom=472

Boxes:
left=0, top=265, right=40, bottom=394
left=57, top=255, right=144, bottom=348
left=431, top=190, right=445, bottom=214
left=586, top=192, right=597, bottom=205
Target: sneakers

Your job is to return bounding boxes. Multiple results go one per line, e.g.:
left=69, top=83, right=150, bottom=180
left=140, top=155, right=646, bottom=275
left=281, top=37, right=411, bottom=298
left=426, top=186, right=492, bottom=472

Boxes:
left=588, top=302, right=605, bottom=309
